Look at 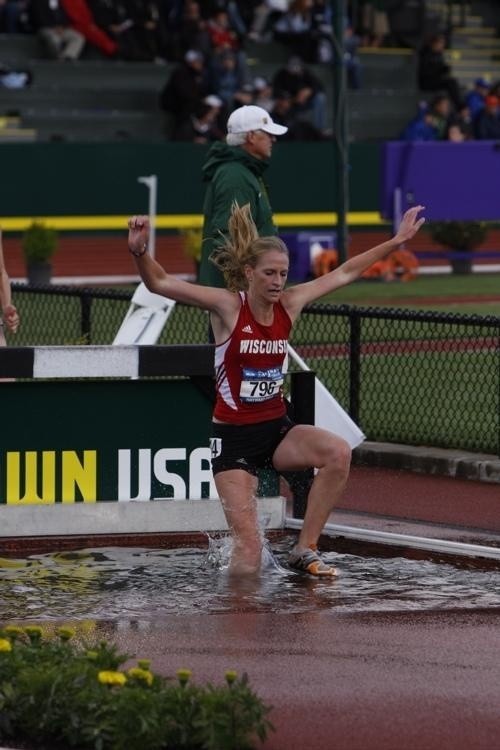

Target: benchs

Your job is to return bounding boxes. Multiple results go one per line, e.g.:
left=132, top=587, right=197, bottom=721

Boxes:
left=0, top=9, right=500, bottom=143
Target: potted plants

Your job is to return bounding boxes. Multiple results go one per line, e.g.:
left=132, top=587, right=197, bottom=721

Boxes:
left=433, top=216, right=488, bottom=273
left=21, top=224, right=59, bottom=285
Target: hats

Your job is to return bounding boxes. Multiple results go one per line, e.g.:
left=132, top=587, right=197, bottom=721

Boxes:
left=227, top=104, right=288, bottom=135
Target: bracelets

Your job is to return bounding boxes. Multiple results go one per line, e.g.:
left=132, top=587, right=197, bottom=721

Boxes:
left=129, top=242, right=147, bottom=258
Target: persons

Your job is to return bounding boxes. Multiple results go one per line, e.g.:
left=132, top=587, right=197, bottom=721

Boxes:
left=124, top=202, right=428, bottom=575
left=196, top=101, right=293, bottom=292
left=3, top=3, right=499, bottom=140
left=1, top=247, right=24, bottom=346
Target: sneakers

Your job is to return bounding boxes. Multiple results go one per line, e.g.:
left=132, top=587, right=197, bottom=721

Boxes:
left=288, top=548, right=337, bottom=580
